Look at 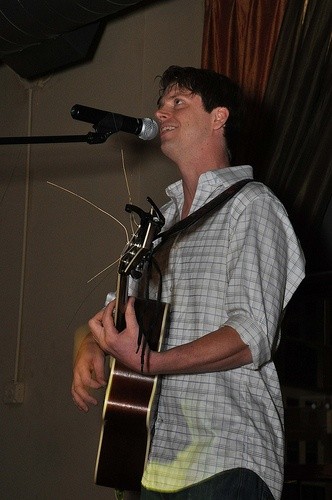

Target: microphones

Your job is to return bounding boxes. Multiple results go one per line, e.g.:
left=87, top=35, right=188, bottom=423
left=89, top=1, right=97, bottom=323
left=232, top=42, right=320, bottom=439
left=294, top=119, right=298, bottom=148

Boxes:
left=71, top=104, right=158, bottom=141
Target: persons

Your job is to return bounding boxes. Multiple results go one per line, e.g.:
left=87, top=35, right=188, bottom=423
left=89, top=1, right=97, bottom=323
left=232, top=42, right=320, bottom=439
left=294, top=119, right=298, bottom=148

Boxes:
left=72, top=64, right=306, bottom=500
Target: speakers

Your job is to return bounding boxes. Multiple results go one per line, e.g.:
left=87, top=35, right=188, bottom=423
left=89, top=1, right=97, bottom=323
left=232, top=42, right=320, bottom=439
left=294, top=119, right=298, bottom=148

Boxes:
left=4, top=17, right=107, bottom=81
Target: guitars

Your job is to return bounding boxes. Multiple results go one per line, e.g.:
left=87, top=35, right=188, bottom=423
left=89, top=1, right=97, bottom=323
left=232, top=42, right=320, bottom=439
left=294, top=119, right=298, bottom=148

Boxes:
left=93, top=197, right=171, bottom=492
